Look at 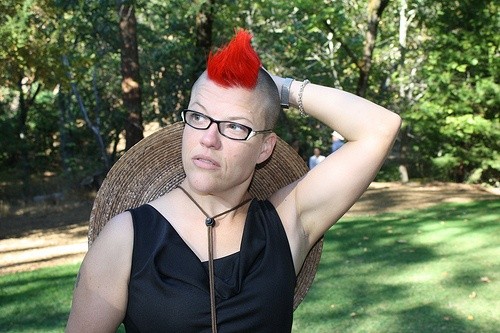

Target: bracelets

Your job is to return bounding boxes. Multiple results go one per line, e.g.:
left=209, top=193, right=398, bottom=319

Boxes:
left=297, top=79, right=310, bottom=120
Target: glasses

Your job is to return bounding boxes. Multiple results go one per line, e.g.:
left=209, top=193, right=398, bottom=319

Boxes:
left=181, top=109, right=274, bottom=141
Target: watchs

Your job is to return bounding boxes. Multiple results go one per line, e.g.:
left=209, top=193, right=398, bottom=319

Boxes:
left=278, top=77, right=298, bottom=110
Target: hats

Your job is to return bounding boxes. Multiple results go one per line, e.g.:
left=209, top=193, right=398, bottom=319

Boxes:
left=88, top=121, right=323, bottom=312
left=331, top=130, right=345, bottom=140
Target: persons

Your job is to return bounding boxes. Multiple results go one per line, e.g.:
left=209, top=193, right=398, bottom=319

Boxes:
left=309, top=147, right=327, bottom=170
left=66, top=27, right=403, bottom=333
left=289, top=137, right=303, bottom=159
left=456, top=162, right=496, bottom=185
